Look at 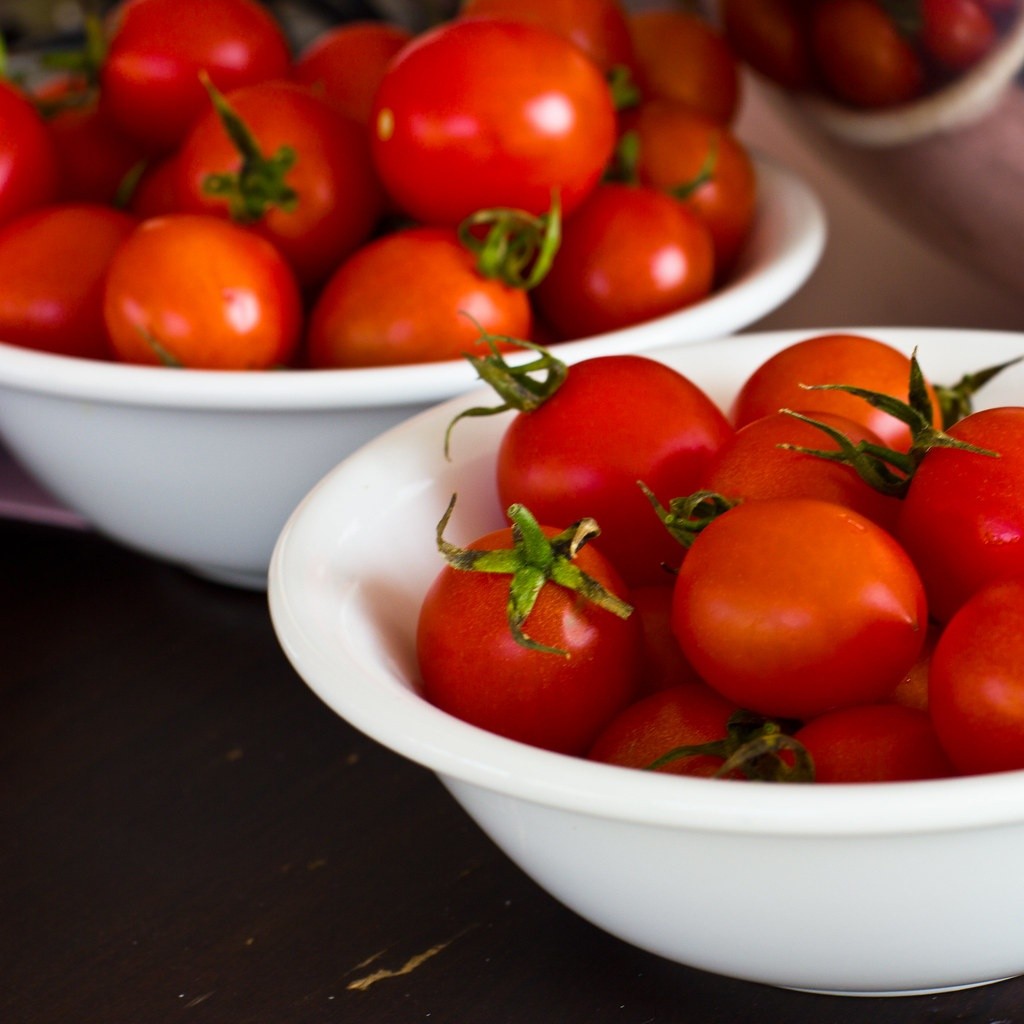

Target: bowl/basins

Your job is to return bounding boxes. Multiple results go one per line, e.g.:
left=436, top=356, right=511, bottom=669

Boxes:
left=268, top=327, right=1024, bottom=993
left=0, top=161, right=823, bottom=587
left=797, top=0, right=1023, bottom=149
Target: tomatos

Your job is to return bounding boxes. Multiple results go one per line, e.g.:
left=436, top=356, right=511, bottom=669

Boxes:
left=417, top=309, right=1024, bottom=782
left=0, top=0, right=1024, bottom=369
